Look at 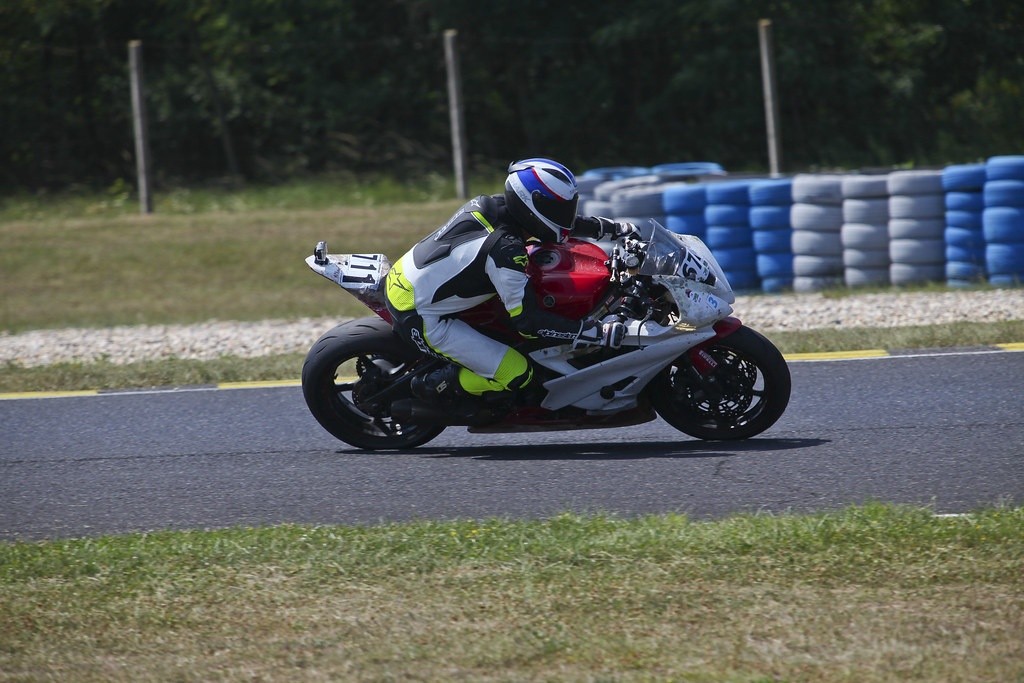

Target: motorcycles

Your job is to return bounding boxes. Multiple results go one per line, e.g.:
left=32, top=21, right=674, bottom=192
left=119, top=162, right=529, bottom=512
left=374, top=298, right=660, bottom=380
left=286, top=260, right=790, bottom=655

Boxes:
left=299, top=213, right=794, bottom=445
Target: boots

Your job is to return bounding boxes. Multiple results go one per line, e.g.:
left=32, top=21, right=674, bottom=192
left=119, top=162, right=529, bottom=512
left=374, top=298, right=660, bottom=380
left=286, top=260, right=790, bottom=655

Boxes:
left=410, top=363, right=481, bottom=419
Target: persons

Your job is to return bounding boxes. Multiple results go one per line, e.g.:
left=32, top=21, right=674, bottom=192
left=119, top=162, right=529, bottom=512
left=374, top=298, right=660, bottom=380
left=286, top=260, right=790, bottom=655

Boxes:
left=382, top=158, right=643, bottom=409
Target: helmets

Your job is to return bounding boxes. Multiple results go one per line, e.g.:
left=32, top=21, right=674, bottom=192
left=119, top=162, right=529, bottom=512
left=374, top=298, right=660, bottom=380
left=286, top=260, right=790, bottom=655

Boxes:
left=503, top=157, right=580, bottom=245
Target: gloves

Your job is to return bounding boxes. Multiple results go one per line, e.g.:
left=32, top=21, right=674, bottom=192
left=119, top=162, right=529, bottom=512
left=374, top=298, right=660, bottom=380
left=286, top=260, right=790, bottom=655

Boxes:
left=590, top=214, right=643, bottom=241
left=572, top=318, right=628, bottom=350
